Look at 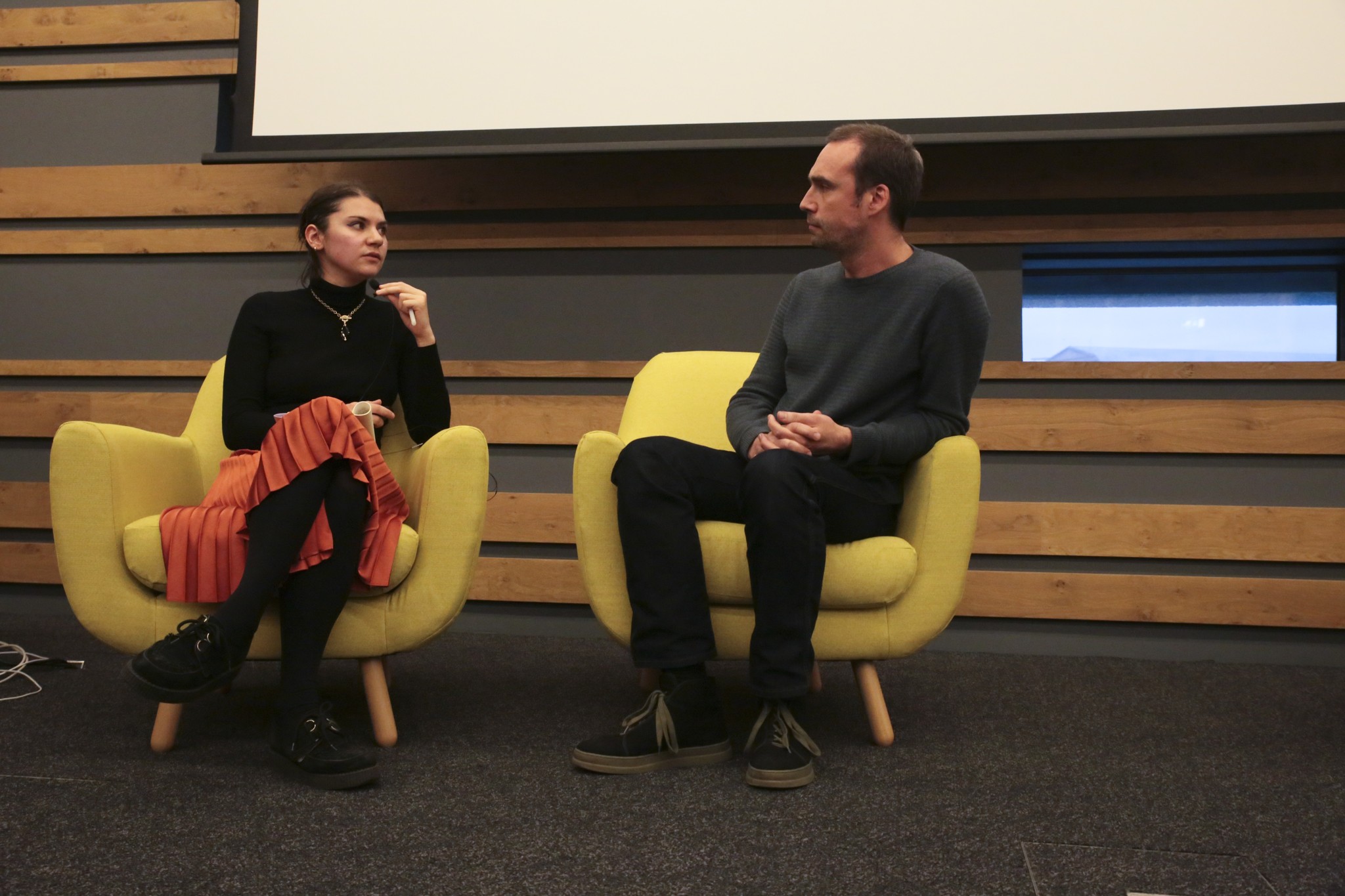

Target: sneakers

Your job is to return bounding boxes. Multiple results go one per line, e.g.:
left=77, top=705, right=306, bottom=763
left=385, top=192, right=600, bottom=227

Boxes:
left=123, top=612, right=247, bottom=704
left=570, top=677, right=731, bottom=774
left=261, top=702, right=380, bottom=790
left=746, top=694, right=821, bottom=788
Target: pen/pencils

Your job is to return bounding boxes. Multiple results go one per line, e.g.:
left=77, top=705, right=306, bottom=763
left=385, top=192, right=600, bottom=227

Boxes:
left=409, top=309, right=416, bottom=326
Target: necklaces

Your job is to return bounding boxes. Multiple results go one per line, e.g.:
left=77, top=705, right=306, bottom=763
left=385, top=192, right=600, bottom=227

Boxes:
left=308, top=282, right=366, bottom=342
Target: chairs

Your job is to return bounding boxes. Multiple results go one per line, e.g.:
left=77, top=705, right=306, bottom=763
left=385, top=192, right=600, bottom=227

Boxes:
left=571, top=350, right=980, bottom=746
left=47, top=356, right=490, bottom=749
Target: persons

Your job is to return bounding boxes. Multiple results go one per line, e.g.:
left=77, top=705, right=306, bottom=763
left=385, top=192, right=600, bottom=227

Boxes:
left=571, top=122, right=989, bottom=787
left=124, top=184, right=451, bottom=791
